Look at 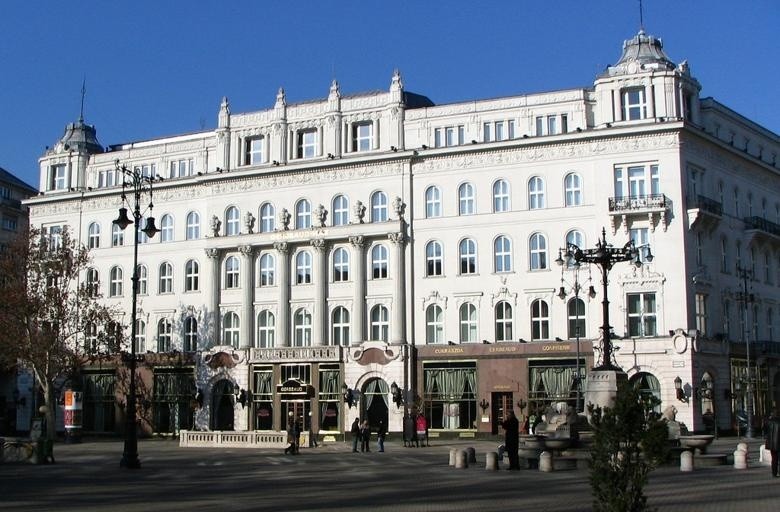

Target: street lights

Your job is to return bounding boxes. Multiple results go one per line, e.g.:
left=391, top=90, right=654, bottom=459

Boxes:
left=734, top=262, right=757, bottom=438
left=555, top=226, right=655, bottom=372
left=556, top=261, right=597, bottom=413
left=112, top=158, right=164, bottom=470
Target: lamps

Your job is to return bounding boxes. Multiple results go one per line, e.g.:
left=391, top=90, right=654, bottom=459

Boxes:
left=191, top=385, right=203, bottom=407
left=391, top=381, right=401, bottom=407
left=674, top=375, right=683, bottom=399
left=233, top=382, right=247, bottom=409
left=340, top=381, right=353, bottom=408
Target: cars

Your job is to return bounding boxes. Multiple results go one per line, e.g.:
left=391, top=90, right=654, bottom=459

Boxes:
left=730, top=408, right=749, bottom=429
left=704, top=413, right=715, bottom=429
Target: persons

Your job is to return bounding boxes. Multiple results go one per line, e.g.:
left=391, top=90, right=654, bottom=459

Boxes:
left=765, top=407, right=779, bottom=478
left=417, top=413, right=427, bottom=447
left=350, top=417, right=359, bottom=453
left=376, top=416, right=386, bottom=453
left=702, top=409, right=714, bottom=432
left=358, top=419, right=373, bottom=453
left=285, top=419, right=295, bottom=455
left=404, top=415, right=415, bottom=448
left=501, top=409, right=520, bottom=471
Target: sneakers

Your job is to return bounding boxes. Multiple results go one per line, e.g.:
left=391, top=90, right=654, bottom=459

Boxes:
left=284, top=449, right=520, bottom=473
